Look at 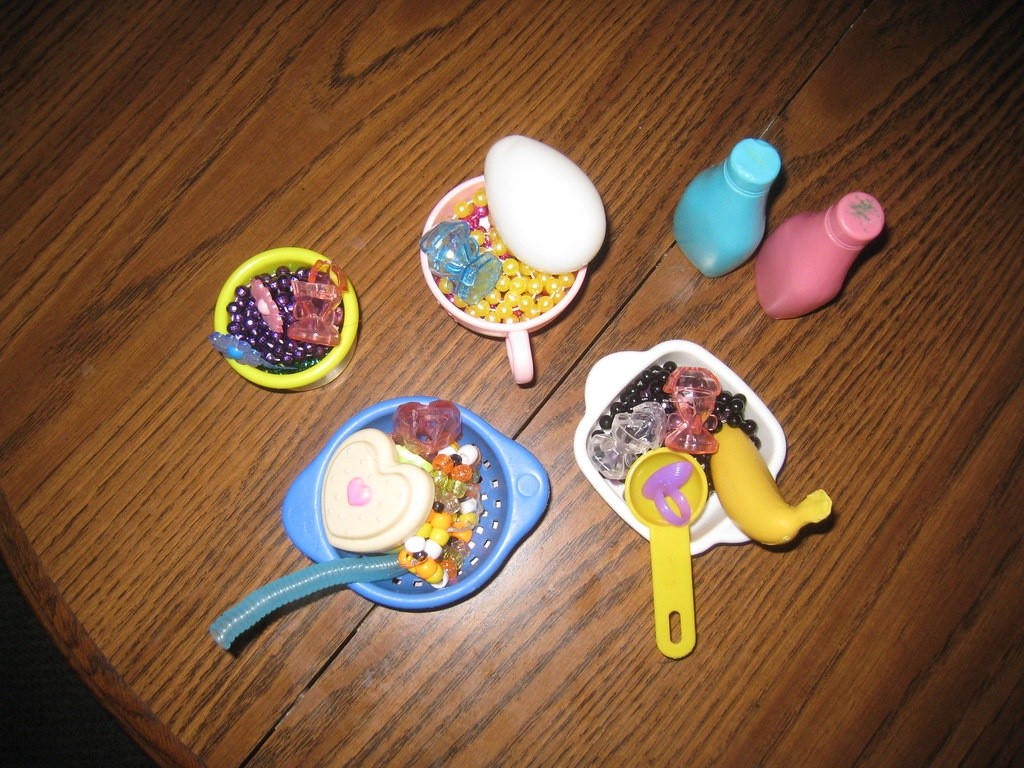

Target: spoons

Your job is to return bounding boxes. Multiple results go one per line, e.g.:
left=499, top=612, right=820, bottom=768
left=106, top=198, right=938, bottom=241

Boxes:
left=626, top=448, right=708, bottom=659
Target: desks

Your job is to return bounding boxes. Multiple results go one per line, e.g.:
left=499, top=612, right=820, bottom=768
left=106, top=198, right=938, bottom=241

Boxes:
left=0, top=0, right=1024, bottom=768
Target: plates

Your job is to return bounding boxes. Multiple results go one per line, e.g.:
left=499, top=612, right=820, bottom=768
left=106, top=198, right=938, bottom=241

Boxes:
left=280, top=396, right=549, bottom=610
left=575, top=340, right=784, bottom=556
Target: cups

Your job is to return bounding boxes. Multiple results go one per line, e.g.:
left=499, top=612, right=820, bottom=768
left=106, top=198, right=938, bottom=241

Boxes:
left=215, top=248, right=358, bottom=390
left=420, top=175, right=586, bottom=384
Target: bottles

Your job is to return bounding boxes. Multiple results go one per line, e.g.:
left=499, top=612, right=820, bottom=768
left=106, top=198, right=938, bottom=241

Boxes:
left=675, top=138, right=886, bottom=322
